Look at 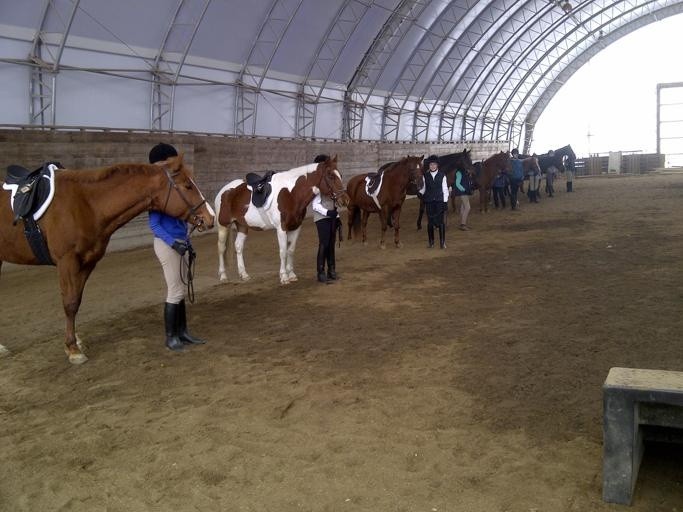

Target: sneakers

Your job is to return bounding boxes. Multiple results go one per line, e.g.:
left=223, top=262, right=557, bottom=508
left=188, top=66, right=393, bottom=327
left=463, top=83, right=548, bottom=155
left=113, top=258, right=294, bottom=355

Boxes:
left=456, top=224, right=469, bottom=231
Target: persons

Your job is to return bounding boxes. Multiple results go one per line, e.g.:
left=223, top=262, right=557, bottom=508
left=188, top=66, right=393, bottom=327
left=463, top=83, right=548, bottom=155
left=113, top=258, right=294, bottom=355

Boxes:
left=311, top=154, right=343, bottom=284
left=454, top=165, right=474, bottom=232
left=491, top=148, right=573, bottom=210
left=417, top=154, right=450, bottom=250
left=148, top=142, right=208, bottom=352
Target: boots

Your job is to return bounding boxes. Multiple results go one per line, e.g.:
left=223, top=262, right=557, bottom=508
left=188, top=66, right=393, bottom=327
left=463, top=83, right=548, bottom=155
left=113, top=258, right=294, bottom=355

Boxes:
left=427, top=224, right=434, bottom=248
left=164, top=302, right=184, bottom=352
left=179, top=300, right=206, bottom=345
left=328, top=265, right=339, bottom=280
left=439, top=224, right=446, bottom=249
left=317, top=265, right=327, bottom=282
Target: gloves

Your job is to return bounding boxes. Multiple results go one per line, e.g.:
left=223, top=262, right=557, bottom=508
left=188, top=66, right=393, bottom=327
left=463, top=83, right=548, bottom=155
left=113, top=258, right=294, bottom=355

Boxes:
left=172, top=241, right=187, bottom=255
left=417, top=192, right=422, bottom=199
left=443, top=202, right=448, bottom=210
left=327, top=209, right=338, bottom=218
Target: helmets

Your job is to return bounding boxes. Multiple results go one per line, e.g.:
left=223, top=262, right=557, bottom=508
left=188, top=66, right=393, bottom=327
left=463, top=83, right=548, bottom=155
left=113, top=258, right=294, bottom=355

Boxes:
left=428, top=155, right=439, bottom=163
left=149, top=142, right=177, bottom=164
left=314, top=155, right=328, bottom=163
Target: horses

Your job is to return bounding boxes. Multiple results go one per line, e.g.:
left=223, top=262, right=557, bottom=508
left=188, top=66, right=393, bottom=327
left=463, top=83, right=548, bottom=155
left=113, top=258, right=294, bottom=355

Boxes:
left=0, top=150, right=216, bottom=366
left=377, top=148, right=477, bottom=233
left=451, top=144, right=576, bottom=214
left=346, top=154, right=425, bottom=250
left=213, top=152, right=351, bottom=285
